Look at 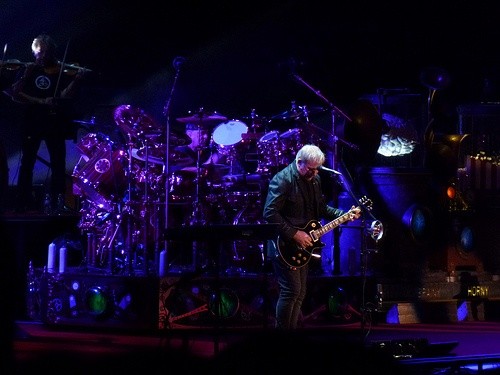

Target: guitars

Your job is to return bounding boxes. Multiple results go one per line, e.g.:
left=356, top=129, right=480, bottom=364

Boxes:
left=275, top=195, right=374, bottom=271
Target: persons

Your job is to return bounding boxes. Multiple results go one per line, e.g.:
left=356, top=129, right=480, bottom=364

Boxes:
left=11, top=35, right=87, bottom=216
left=171, top=124, right=218, bottom=175
left=263, top=144, right=359, bottom=330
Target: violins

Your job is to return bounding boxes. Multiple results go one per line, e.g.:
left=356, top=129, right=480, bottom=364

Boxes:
left=0, top=58, right=36, bottom=70
left=44, top=58, right=104, bottom=79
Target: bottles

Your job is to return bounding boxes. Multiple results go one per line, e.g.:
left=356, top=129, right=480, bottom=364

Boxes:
left=158, top=250, right=167, bottom=274
left=47, top=240, right=56, bottom=269
left=59, top=245, right=68, bottom=273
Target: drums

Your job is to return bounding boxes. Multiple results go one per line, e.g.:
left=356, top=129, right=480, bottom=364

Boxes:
left=79, top=128, right=279, bottom=273
left=261, top=128, right=303, bottom=168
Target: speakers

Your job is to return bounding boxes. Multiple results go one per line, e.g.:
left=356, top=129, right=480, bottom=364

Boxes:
left=207, top=331, right=377, bottom=374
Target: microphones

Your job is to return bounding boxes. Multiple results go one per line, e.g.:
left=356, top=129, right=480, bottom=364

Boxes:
left=317, top=165, right=342, bottom=175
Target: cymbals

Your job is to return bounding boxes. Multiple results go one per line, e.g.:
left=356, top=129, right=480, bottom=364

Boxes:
left=212, top=121, right=249, bottom=146
left=176, top=114, right=226, bottom=124
left=74, top=117, right=97, bottom=129
left=239, top=114, right=266, bottom=122
left=270, top=107, right=312, bottom=120
left=114, top=105, right=161, bottom=140
